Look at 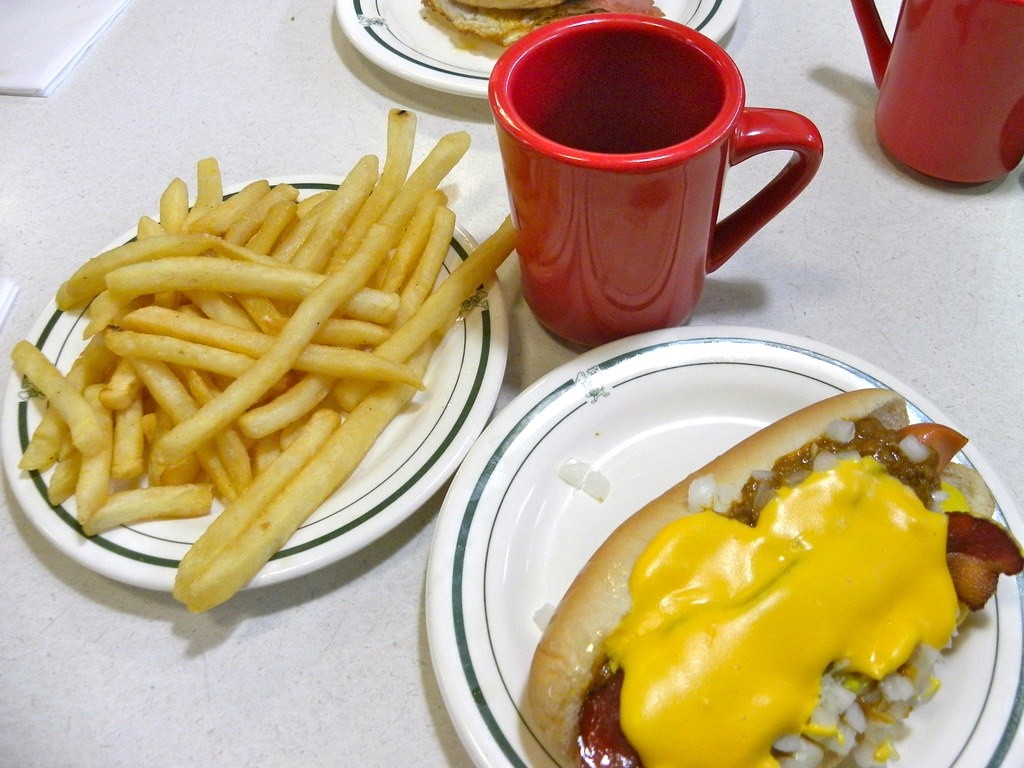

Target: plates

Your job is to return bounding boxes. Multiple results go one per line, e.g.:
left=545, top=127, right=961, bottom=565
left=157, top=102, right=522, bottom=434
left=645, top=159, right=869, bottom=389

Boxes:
left=425, top=326, right=1024, bottom=768
left=0, top=175, right=508, bottom=592
left=336, top=0, right=748, bottom=99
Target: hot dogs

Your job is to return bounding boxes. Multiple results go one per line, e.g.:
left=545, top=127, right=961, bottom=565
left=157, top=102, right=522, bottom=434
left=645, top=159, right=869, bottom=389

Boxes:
left=526, top=388, right=1024, bottom=768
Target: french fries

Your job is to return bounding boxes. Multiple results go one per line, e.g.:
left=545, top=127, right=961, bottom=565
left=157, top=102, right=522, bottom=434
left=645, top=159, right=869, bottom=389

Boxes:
left=8, top=105, right=520, bottom=614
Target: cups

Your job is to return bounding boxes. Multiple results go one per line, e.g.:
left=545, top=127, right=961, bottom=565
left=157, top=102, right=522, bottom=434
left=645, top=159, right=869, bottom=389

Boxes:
left=851, top=0, right=1024, bottom=191
left=488, top=13, right=823, bottom=347
